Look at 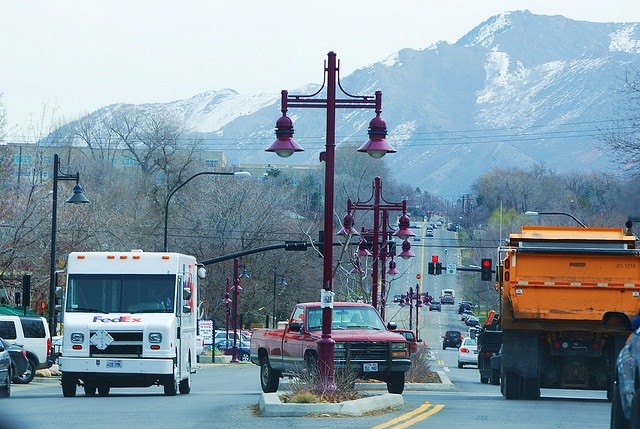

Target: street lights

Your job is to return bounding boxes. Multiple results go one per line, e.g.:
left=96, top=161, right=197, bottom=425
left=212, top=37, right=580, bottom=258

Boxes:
left=355, top=227, right=399, bottom=277
left=49, top=153, right=90, bottom=338
left=230, top=257, right=242, bottom=361
left=350, top=209, right=415, bottom=321
left=163, top=171, right=251, bottom=254
left=265, top=51, right=396, bottom=390
left=344, top=176, right=409, bottom=310
left=223, top=276, right=231, bottom=353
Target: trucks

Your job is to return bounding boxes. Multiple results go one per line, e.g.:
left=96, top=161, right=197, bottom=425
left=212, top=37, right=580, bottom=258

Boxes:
left=441, top=289, right=455, bottom=297
left=53, top=248, right=208, bottom=396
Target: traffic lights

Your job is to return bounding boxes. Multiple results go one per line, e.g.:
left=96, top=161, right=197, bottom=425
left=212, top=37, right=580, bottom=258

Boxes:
left=40, top=303, right=46, bottom=311
left=428, top=262, right=434, bottom=274
left=432, top=255, right=438, bottom=262
left=436, top=263, right=442, bottom=274
left=481, top=258, right=491, bottom=281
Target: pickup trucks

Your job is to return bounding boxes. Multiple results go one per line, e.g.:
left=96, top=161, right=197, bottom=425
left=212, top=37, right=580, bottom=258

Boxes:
left=250, top=301, right=411, bottom=394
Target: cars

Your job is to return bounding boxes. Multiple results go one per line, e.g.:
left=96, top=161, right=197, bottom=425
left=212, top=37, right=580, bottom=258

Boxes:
left=412, top=234, right=423, bottom=243
left=426, top=221, right=435, bottom=231
left=466, top=316, right=480, bottom=326
left=429, top=301, right=441, bottom=312
left=413, top=223, right=422, bottom=230
left=215, top=339, right=250, bottom=361
left=439, top=294, right=455, bottom=305
left=458, top=338, right=478, bottom=367
left=393, top=294, right=401, bottom=302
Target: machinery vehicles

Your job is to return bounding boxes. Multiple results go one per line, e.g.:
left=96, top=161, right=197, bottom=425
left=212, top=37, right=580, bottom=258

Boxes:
left=486, top=221, right=639, bottom=399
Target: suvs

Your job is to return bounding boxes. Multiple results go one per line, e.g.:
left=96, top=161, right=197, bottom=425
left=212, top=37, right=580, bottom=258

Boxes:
left=0, top=316, right=53, bottom=384
left=437, top=217, right=444, bottom=223
left=441, top=331, right=462, bottom=349
left=426, top=229, right=433, bottom=236
